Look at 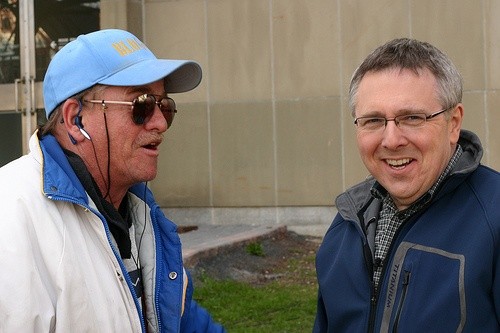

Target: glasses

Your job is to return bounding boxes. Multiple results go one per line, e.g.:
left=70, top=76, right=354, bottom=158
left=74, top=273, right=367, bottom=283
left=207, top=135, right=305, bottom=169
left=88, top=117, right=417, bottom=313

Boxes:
left=81, top=93, right=177, bottom=129
left=352, top=104, right=455, bottom=133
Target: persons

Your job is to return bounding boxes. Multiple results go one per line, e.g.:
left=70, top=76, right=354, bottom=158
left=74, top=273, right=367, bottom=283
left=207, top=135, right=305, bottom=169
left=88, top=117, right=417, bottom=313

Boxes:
left=308, top=37, right=500, bottom=332
left=0, top=28, right=229, bottom=333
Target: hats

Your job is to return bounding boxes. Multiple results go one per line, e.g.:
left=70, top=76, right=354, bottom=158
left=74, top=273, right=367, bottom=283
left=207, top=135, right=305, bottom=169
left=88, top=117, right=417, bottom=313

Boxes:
left=42, top=30, right=202, bottom=119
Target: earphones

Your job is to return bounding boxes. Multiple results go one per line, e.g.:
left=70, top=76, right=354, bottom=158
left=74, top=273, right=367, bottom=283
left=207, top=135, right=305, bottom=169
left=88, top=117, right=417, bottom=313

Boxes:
left=75, top=117, right=91, bottom=140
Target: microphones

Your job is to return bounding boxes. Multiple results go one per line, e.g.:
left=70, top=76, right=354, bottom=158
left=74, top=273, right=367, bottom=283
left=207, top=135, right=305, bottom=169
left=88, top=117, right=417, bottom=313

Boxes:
left=373, top=258, right=381, bottom=271
